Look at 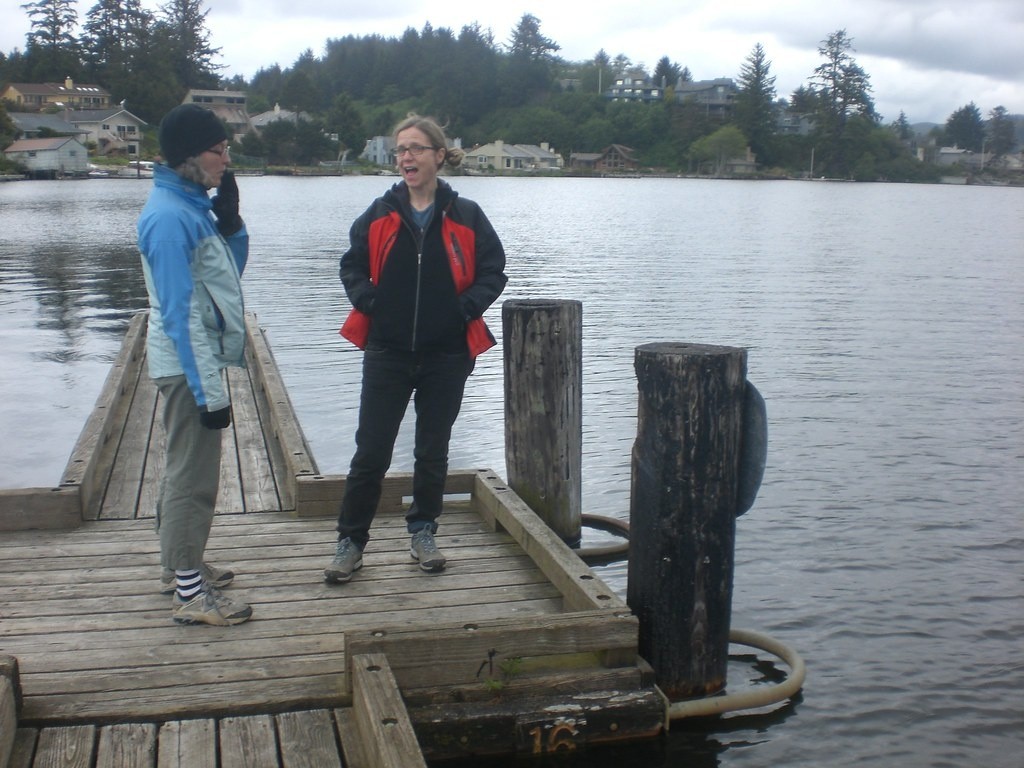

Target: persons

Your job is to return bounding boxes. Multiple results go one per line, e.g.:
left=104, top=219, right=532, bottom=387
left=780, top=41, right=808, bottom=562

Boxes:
left=136, top=102, right=253, bottom=626
left=324, top=112, right=509, bottom=584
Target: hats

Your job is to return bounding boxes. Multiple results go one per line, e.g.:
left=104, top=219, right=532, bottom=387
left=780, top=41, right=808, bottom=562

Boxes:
left=158, top=103, right=227, bottom=167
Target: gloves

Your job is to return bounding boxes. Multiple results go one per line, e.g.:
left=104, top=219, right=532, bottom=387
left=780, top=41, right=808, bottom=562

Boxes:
left=201, top=406, right=230, bottom=428
left=209, top=172, right=240, bottom=221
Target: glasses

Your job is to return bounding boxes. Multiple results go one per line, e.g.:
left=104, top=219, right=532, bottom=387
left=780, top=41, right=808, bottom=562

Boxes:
left=205, top=145, right=231, bottom=158
left=390, top=144, right=438, bottom=156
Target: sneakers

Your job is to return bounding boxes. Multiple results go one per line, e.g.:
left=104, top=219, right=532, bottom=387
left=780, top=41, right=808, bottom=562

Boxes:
left=172, top=580, right=253, bottom=626
left=158, top=563, right=234, bottom=594
left=410, top=523, right=447, bottom=571
left=323, top=537, right=363, bottom=582
